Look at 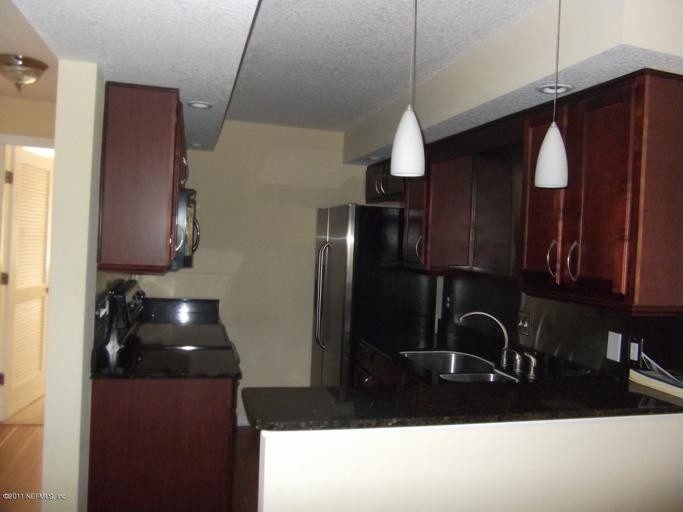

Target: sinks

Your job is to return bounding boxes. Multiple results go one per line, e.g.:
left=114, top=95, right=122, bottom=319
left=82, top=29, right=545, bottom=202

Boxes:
left=438, top=369, right=518, bottom=383
left=396, top=346, right=495, bottom=374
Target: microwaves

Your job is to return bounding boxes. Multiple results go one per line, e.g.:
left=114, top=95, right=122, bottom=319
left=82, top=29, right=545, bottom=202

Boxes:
left=177, top=185, right=201, bottom=268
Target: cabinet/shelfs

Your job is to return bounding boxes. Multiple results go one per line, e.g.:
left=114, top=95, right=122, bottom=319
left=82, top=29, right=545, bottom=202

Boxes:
left=363, top=160, right=406, bottom=202
left=401, top=157, right=429, bottom=270
left=523, top=82, right=638, bottom=299
left=349, top=345, right=405, bottom=386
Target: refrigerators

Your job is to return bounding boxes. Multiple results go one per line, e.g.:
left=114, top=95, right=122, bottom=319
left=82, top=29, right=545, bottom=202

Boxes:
left=310, top=205, right=403, bottom=390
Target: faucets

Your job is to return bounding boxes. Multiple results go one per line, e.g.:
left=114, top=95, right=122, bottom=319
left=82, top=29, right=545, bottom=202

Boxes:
left=458, top=310, right=509, bottom=367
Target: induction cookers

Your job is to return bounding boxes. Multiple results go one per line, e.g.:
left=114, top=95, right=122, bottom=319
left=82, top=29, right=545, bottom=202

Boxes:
left=119, top=321, right=231, bottom=350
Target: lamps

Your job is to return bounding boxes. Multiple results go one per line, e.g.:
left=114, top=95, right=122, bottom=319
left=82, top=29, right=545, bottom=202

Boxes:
left=387, top=2, right=428, bottom=180
left=533, top=1, right=570, bottom=189
left=0, top=53, right=49, bottom=94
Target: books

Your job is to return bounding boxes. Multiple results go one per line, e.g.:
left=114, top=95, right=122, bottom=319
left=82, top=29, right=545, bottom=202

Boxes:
left=627, top=379, right=683, bottom=409
left=628, top=350, right=682, bottom=399
